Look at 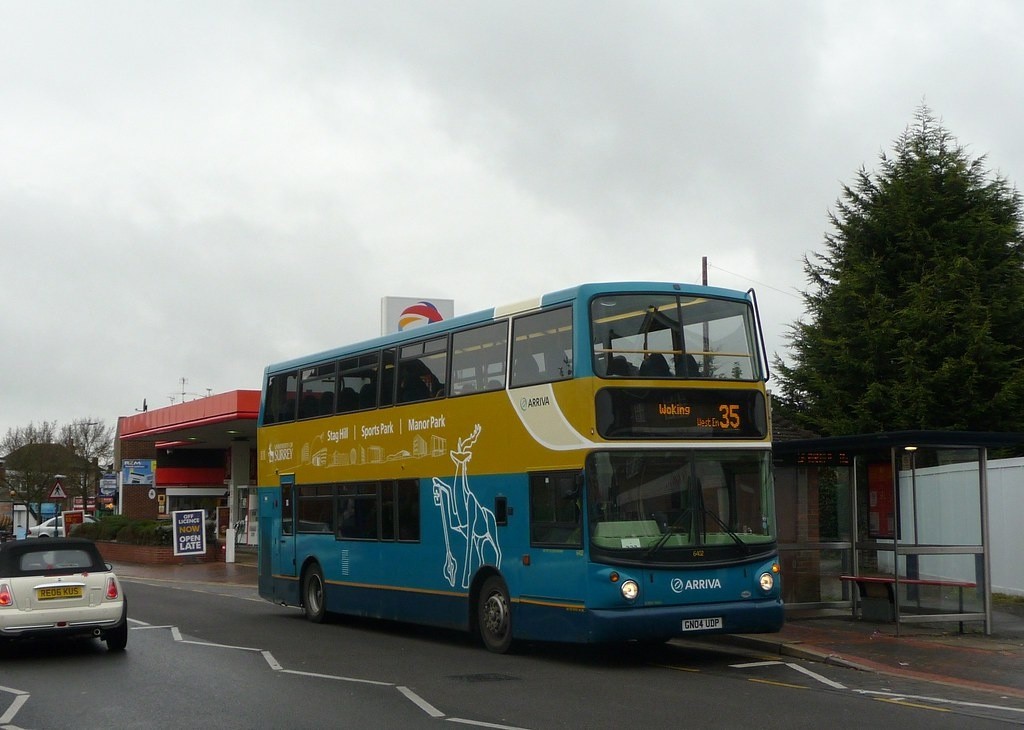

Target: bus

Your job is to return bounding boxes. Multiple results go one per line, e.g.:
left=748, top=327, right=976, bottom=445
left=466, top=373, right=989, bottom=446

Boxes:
left=256, top=281, right=785, bottom=655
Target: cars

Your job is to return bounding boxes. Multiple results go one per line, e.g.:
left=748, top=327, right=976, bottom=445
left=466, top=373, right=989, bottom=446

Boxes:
left=0, top=537, right=128, bottom=654
left=27, top=514, right=101, bottom=538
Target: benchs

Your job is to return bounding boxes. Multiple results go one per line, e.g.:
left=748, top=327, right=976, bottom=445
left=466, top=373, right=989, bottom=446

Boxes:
left=840, top=574, right=977, bottom=631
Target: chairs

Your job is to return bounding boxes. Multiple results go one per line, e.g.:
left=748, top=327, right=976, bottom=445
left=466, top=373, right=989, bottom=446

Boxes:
left=282, top=352, right=704, bottom=422
left=23, top=554, right=47, bottom=569
left=54, top=552, right=86, bottom=565
